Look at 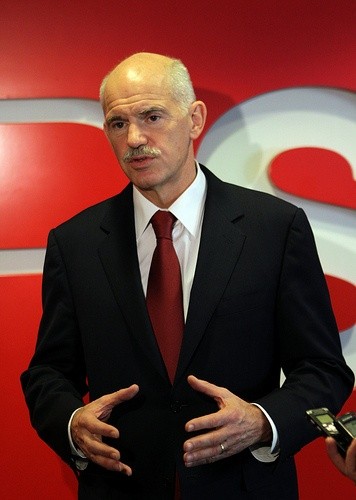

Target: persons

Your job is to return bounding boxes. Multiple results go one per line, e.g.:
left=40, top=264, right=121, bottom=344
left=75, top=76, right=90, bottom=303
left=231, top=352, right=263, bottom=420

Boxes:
left=19, top=53, right=355, bottom=500
left=324, top=434, right=356, bottom=481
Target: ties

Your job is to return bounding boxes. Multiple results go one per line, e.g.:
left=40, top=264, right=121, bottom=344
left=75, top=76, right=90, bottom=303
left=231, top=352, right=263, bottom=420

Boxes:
left=145, top=210, right=184, bottom=386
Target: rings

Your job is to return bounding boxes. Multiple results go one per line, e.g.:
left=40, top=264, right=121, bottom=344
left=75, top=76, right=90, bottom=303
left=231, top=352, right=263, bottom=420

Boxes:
left=219, top=443, right=225, bottom=455
